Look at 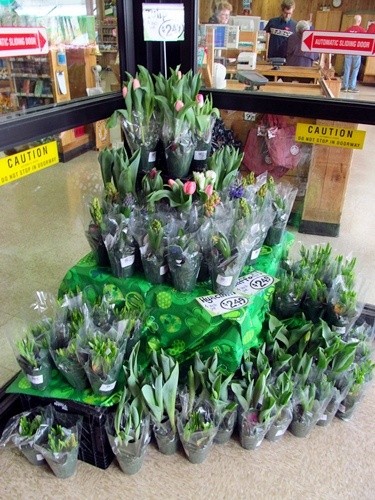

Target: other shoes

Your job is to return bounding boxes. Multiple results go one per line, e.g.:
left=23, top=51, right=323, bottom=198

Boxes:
left=341, top=87, right=347, bottom=92
left=348, top=87, right=360, bottom=93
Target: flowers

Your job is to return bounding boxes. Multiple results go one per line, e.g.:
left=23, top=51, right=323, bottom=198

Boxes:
left=85, top=66, right=298, bottom=292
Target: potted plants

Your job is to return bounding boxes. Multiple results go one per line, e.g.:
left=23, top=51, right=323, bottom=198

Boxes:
left=0, top=244, right=375, bottom=479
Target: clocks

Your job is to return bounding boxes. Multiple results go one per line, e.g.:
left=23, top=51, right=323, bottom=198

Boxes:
left=331, top=0, right=343, bottom=8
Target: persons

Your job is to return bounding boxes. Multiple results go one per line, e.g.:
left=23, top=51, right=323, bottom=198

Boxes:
left=286, top=20, right=320, bottom=66
left=341, top=15, right=365, bottom=92
left=209, top=2, right=233, bottom=24
left=263, top=0, right=298, bottom=59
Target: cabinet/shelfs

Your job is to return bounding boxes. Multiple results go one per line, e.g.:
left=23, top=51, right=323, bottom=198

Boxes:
left=316, top=10, right=375, bottom=83
left=0, top=40, right=112, bottom=163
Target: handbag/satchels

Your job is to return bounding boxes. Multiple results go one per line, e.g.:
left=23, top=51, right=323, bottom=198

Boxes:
left=240, top=113, right=291, bottom=181
left=265, top=113, right=303, bottom=169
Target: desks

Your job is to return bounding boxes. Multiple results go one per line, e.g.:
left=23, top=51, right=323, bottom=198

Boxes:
left=3, top=231, right=294, bottom=407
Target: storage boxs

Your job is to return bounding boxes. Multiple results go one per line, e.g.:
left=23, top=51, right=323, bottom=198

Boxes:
left=19, top=394, right=115, bottom=470
